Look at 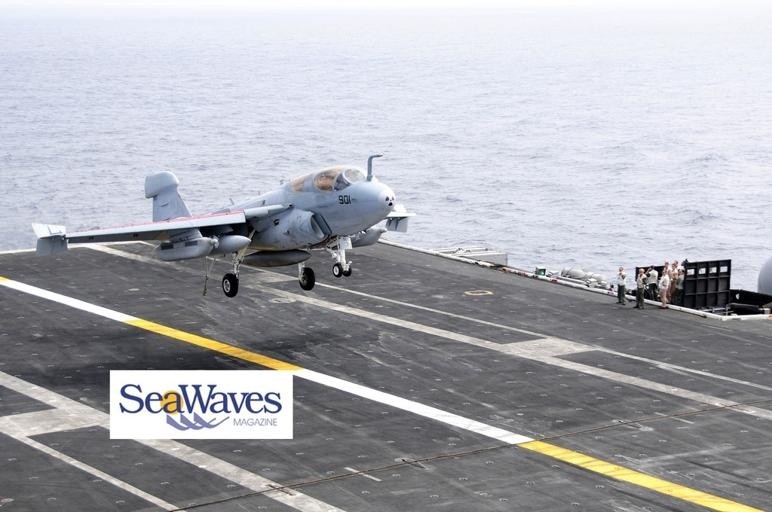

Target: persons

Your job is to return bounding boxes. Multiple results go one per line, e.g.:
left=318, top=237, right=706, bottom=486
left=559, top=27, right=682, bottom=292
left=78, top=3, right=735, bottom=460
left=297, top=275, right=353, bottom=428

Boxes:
left=644, top=260, right=685, bottom=309
left=615, top=266, right=627, bottom=306
left=632, top=268, right=647, bottom=311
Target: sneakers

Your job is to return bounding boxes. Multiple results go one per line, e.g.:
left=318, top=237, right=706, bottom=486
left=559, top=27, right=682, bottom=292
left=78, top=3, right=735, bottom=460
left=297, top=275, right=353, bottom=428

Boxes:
left=658, top=305, right=669, bottom=309
left=633, top=305, right=644, bottom=310
left=616, top=301, right=626, bottom=305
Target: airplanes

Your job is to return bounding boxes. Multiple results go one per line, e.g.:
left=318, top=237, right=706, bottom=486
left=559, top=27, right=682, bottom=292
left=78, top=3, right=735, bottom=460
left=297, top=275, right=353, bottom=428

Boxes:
left=33, top=153, right=418, bottom=299
